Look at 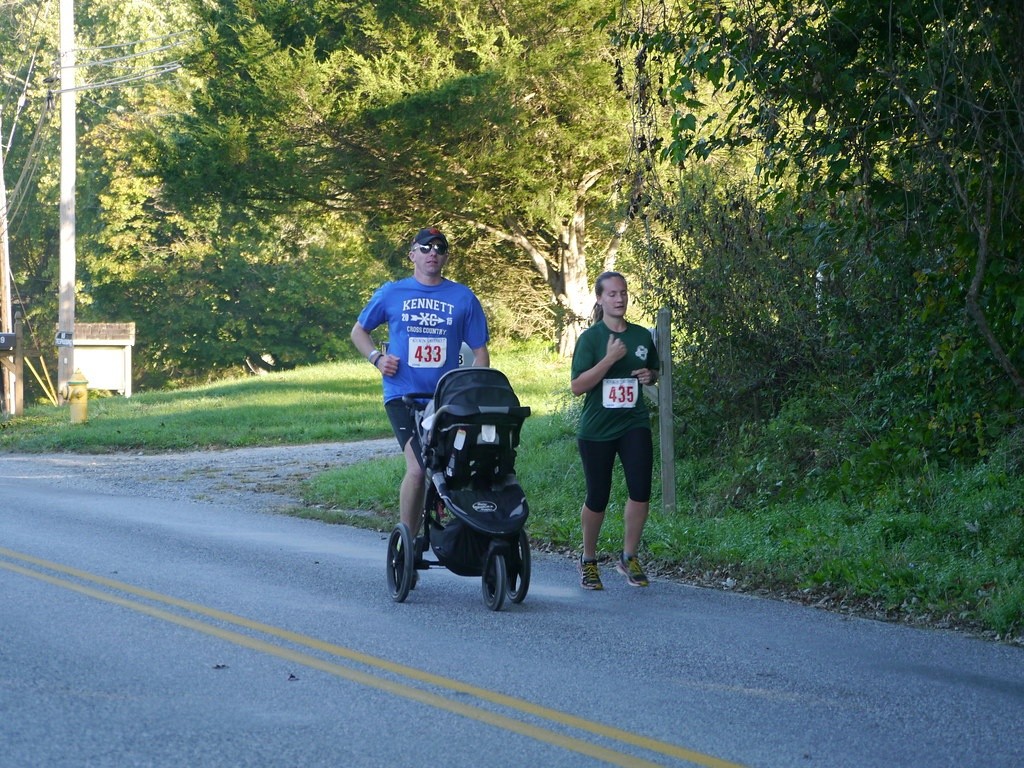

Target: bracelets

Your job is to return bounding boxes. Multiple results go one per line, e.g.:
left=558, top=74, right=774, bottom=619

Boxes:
left=374, top=354, right=384, bottom=366
left=368, top=350, right=379, bottom=361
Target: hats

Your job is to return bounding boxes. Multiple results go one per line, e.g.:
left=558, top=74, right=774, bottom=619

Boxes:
left=413, top=228, right=448, bottom=247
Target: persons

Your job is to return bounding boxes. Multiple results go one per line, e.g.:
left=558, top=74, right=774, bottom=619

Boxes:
left=351, top=228, right=490, bottom=590
left=571, top=273, right=660, bottom=589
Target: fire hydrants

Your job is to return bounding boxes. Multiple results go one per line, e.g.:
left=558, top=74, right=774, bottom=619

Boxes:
left=58, top=367, right=90, bottom=425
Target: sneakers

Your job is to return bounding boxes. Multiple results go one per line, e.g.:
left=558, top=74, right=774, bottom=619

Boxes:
left=617, top=550, right=649, bottom=587
left=577, top=553, right=604, bottom=590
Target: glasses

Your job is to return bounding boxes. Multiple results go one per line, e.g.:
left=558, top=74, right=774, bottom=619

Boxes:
left=412, top=243, right=447, bottom=255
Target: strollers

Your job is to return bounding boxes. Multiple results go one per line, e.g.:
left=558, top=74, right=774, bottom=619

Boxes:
left=384, top=365, right=534, bottom=611
